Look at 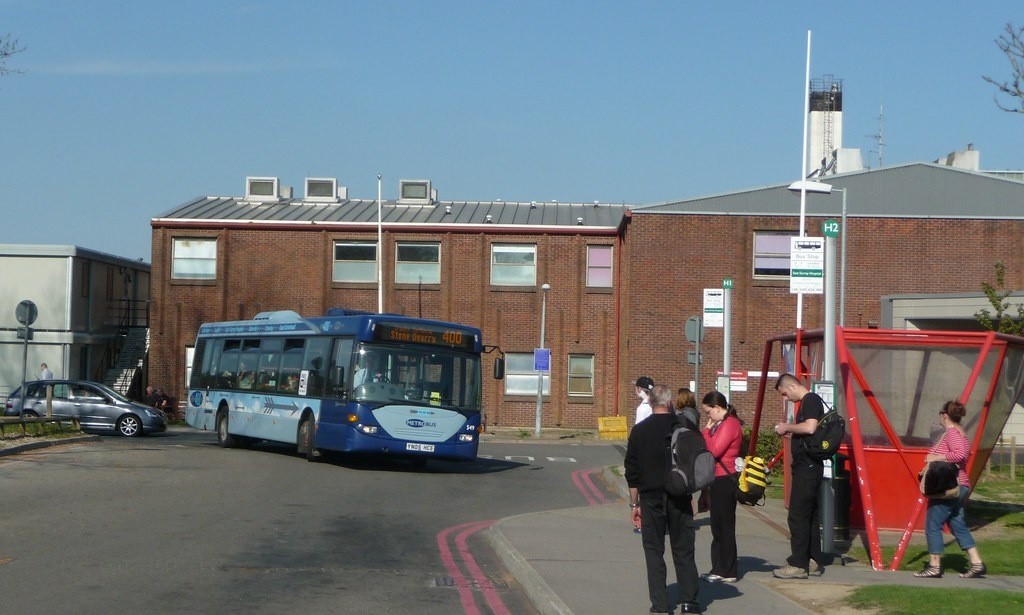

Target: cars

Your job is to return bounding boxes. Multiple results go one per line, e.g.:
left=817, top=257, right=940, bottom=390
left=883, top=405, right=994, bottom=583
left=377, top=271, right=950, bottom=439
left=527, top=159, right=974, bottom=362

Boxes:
left=5, top=380, right=168, bottom=437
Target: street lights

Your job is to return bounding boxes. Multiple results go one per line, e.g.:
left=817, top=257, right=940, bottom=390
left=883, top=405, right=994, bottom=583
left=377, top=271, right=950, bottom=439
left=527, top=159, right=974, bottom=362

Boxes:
left=786, top=181, right=847, bottom=327
left=534, top=284, right=551, bottom=439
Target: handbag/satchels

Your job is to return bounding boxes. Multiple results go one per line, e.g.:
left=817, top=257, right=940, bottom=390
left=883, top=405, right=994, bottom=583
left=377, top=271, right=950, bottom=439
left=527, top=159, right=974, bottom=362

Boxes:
left=920, top=468, right=960, bottom=500
left=734, top=456, right=770, bottom=507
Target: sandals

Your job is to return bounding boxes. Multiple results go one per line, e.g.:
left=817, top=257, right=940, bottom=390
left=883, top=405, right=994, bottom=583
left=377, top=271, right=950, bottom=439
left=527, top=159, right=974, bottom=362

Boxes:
left=914, top=564, right=942, bottom=578
left=959, top=562, right=986, bottom=578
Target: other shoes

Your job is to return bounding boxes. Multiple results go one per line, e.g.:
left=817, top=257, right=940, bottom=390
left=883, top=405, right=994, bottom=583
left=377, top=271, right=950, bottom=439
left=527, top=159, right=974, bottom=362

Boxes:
left=682, top=602, right=700, bottom=615
left=701, top=572, right=737, bottom=583
left=649, top=607, right=669, bottom=615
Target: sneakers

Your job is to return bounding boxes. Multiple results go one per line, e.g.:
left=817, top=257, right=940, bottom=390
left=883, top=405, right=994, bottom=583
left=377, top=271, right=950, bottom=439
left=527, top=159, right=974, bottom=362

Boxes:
left=773, top=561, right=808, bottom=579
left=807, top=559, right=820, bottom=576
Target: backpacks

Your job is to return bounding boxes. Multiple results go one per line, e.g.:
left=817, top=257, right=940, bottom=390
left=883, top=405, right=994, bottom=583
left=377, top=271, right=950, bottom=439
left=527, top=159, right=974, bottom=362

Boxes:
left=800, top=393, right=845, bottom=461
left=665, top=415, right=715, bottom=497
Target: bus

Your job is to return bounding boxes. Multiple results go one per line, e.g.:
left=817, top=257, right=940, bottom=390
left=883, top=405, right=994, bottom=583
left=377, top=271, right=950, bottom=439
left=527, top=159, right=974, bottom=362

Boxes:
left=185, top=307, right=506, bottom=470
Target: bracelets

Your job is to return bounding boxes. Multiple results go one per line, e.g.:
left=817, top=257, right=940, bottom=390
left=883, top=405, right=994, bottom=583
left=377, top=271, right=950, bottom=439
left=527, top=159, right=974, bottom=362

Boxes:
left=629, top=503, right=639, bottom=507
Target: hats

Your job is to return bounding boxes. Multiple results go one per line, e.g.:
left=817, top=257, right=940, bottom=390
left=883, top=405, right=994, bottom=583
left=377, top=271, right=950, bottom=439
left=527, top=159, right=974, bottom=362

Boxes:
left=632, top=377, right=654, bottom=390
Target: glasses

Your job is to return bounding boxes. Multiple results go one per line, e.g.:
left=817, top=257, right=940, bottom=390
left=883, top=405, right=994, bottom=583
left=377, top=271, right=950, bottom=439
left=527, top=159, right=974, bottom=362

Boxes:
left=706, top=408, right=712, bottom=414
left=939, top=411, right=947, bottom=415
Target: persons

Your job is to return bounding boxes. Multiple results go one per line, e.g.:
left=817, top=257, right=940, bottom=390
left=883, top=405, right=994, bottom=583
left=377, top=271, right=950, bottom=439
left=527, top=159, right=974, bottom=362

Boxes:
left=635, top=377, right=655, bottom=424
left=701, top=391, right=743, bottom=585
left=143, top=385, right=172, bottom=413
left=355, top=358, right=382, bottom=388
left=40, top=363, right=53, bottom=380
left=624, top=385, right=700, bottom=615
left=674, top=388, right=701, bottom=427
left=239, top=370, right=255, bottom=389
left=772, top=374, right=823, bottom=579
left=913, top=401, right=987, bottom=578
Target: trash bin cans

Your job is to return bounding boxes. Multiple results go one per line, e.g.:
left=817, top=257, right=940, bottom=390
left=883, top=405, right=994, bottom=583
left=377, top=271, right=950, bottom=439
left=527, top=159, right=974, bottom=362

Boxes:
left=597, top=416, right=628, bottom=441
left=818, top=452, right=851, bottom=541
